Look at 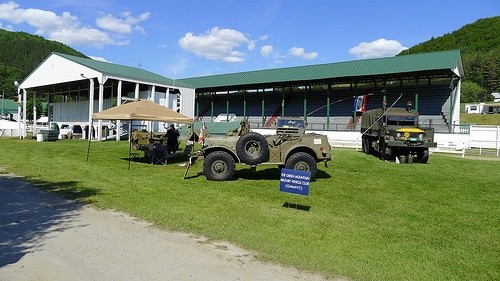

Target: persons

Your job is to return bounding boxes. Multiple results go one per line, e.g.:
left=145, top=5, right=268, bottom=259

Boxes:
left=183, top=126, right=193, bottom=155
left=131, top=129, right=143, bottom=150
left=2, top=114, right=9, bottom=119
left=406, top=98, right=412, bottom=112
left=166, top=123, right=180, bottom=154
left=238, top=118, right=245, bottom=136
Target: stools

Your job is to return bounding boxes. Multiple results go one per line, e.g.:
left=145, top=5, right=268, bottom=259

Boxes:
left=131, top=153, right=140, bottom=160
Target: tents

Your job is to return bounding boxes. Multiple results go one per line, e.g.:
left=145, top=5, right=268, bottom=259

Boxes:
left=86, top=100, right=196, bottom=170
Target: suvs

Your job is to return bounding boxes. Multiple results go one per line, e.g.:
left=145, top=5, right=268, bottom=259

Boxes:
left=198, top=117, right=331, bottom=182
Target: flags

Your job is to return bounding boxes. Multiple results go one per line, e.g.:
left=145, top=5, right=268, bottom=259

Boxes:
left=352, top=94, right=367, bottom=112
left=198, top=126, right=205, bottom=144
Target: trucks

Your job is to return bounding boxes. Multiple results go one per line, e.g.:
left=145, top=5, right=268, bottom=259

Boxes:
left=274, top=117, right=308, bottom=138
left=361, top=100, right=438, bottom=164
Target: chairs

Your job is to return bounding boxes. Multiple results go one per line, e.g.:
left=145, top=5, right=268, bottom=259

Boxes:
left=145, top=139, right=194, bottom=166
